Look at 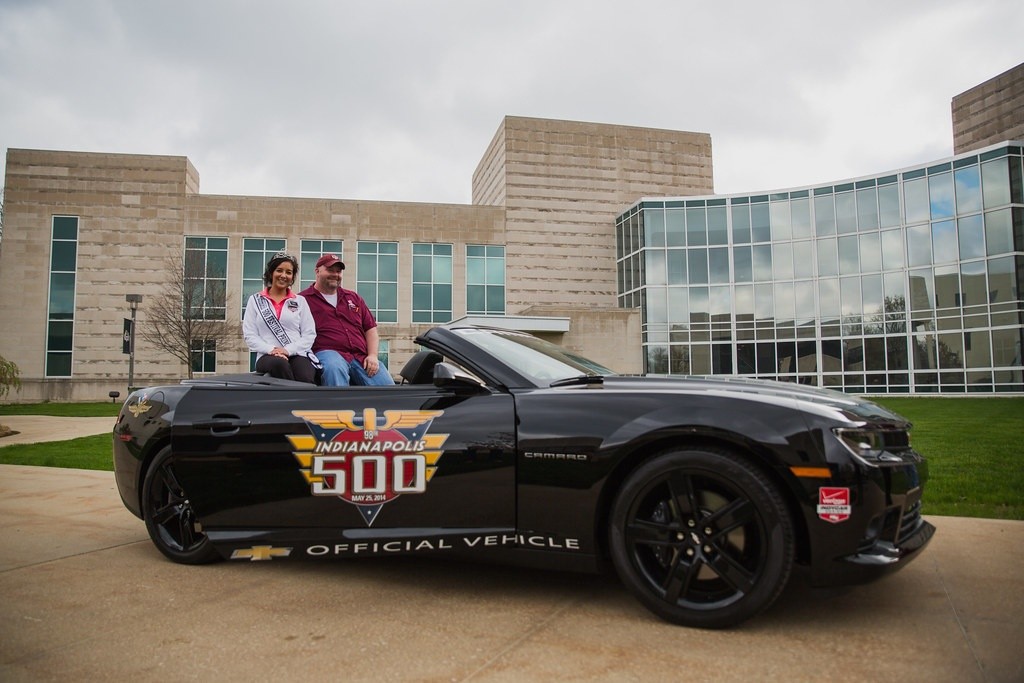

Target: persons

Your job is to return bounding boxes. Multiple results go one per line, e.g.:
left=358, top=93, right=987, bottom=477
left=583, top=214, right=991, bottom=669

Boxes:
left=243, top=252, right=317, bottom=382
left=295, top=255, right=396, bottom=387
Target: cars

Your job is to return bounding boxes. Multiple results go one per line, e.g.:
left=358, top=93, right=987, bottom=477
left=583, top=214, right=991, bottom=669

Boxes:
left=112, top=324, right=938, bottom=629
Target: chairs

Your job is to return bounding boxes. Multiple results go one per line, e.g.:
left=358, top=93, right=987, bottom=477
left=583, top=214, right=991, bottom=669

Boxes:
left=399, top=351, right=444, bottom=384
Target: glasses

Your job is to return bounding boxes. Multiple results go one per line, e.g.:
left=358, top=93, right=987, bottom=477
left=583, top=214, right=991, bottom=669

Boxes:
left=273, top=251, right=295, bottom=263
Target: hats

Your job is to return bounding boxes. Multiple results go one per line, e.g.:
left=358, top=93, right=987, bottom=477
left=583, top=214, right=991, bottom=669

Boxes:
left=316, top=254, right=345, bottom=270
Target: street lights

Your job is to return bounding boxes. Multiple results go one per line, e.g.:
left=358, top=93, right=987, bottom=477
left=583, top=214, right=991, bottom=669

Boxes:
left=125, top=293, right=142, bottom=386
